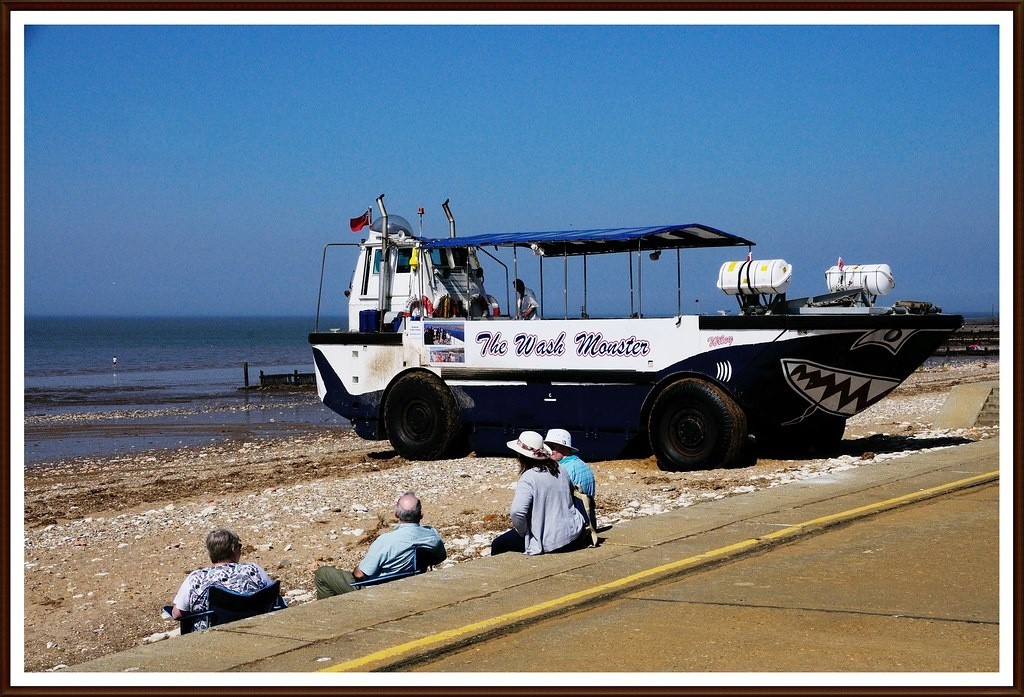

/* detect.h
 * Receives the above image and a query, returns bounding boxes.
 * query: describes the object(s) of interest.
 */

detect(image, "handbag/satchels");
[574,484,599,548]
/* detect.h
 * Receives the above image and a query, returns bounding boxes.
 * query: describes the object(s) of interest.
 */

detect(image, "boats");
[306,192,966,460]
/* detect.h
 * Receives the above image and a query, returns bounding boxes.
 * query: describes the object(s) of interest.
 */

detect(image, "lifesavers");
[404,294,433,319]
[465,293,500,317]
[432,293,458,319]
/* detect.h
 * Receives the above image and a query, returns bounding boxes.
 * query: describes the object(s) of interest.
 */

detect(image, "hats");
[506,431,552,459]
[543,429,579,452]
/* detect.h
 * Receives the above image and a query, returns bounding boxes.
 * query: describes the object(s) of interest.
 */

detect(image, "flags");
[349,210,369,232]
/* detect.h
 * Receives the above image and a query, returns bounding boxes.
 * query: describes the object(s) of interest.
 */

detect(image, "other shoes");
[163,606,173,619]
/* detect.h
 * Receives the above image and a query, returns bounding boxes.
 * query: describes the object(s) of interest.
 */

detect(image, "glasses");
[238,543,242,549]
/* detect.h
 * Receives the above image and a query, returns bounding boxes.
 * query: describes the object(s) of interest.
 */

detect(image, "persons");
[512,278,540,321]
[490,431,586,557]
[172,529,288,633]
[314,494,447,602]
[543,429,595,534]
